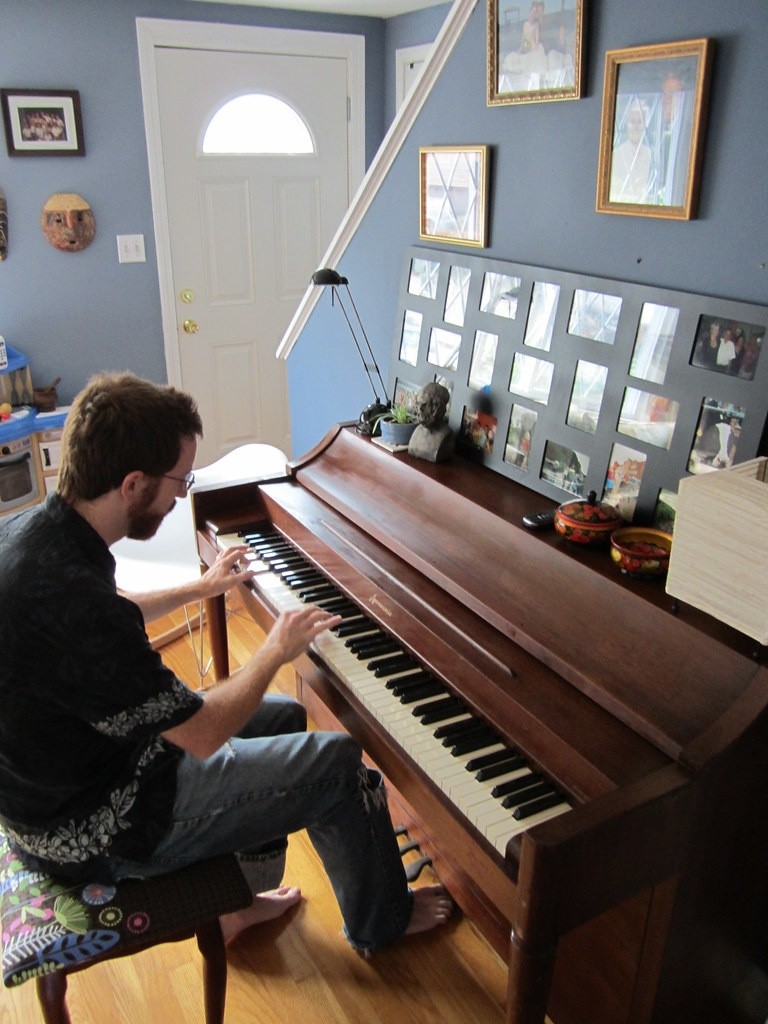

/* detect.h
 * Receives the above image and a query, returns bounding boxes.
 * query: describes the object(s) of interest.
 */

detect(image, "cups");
[33,387,56,412]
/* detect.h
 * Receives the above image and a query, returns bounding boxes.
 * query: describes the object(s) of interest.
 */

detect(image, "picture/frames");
[0,88,86,156]
[418,146,489,248]
[596,38,709,221]
[486,0,583,107]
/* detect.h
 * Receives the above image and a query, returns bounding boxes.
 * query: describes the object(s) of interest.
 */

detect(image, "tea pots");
[356,397,392,435]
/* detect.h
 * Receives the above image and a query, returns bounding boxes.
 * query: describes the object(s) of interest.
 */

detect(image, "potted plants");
[368,388,423,446]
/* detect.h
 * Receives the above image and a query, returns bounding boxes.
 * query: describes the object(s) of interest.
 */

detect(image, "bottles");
[0,335,8,370]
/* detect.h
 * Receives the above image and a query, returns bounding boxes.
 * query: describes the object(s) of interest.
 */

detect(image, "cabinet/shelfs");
[0,347,72,520]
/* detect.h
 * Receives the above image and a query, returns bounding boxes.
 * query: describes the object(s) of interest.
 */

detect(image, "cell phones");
[522,509,557,528]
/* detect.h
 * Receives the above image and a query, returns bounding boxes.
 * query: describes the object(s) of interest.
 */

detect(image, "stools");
[0,823,253,1024]
[108,442,289,696]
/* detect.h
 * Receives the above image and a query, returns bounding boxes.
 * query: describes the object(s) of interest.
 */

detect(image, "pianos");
[185,411,768,1023]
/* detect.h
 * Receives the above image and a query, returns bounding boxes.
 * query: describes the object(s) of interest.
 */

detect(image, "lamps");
[312,268,391,435]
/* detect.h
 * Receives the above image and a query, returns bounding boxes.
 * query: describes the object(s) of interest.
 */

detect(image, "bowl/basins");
[555,490,620,543]
[610,527,672,573]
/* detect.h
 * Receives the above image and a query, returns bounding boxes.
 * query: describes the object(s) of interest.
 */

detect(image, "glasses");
[141,470,195,490]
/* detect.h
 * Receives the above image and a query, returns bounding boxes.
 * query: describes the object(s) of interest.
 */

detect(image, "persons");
[0,373,451,955]
[610,75,688,207]
[514,431,530,466]
[698,325,762,379]
[472,422,490,454]
[501,0,545,72]
[699,417,740,468]
[559,459,576,493]
[21,110,64,140]
